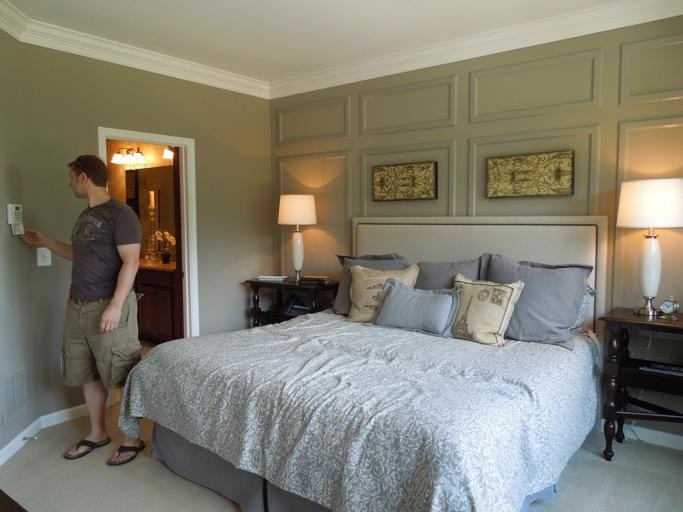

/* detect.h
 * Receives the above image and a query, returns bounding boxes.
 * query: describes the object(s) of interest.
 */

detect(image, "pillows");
[415,253,488,290]
[366,276,463,341]
[571,285,598,335]
[449,274,524,347]
[479,252,593,352]
[334,252,412,318]
[347,265,421,324]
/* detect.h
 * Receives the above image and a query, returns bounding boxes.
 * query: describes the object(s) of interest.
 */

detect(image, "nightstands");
[247,278,338,329]
[597,307,682,461]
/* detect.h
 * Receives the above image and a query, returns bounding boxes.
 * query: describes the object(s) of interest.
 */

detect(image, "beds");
[118,215,609,512]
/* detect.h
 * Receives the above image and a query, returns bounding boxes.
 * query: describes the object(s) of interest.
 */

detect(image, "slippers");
[63,435,110,459]
[106,439,145,465]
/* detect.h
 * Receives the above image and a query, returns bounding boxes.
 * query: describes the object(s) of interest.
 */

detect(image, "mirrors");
[125,165,174,262]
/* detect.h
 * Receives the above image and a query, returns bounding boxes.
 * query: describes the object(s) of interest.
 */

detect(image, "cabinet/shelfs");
[134,268,182,342]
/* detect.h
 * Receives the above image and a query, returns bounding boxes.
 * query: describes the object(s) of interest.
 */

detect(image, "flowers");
[154,230,176,251]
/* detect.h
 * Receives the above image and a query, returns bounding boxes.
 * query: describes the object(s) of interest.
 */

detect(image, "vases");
[162,251,170,265]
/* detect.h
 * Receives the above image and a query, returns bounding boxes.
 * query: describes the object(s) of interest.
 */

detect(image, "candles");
[149,191,156,207]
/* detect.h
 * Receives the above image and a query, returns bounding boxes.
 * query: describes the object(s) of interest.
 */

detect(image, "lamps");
[277,194,317,281]
[111,143,142,165]
[614,178,683,319]
[162,147,175,162]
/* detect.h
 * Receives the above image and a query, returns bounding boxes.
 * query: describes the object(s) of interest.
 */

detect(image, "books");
[299,275,331,286]
[637,360,683,377]
[256,274,289,282]
[289,305,312,316]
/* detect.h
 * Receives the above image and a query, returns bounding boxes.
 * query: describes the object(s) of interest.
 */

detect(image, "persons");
[19,154,145,465]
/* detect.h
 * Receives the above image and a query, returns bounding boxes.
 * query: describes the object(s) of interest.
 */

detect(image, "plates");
[256,275,288,282]
